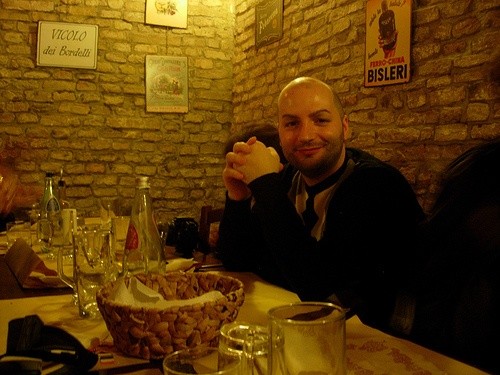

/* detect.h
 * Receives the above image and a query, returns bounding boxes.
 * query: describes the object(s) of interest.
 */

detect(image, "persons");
[209,123,289,270]
[218,77,429,338]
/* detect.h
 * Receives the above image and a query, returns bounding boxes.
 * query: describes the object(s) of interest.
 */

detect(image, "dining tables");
[0,223,491,375]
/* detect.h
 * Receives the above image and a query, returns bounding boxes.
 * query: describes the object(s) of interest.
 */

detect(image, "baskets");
[96,272,245,360]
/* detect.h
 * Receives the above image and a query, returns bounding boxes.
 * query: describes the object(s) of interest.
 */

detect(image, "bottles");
[40,172,63,253]
[58,181,78,224]
[123,176,167,278]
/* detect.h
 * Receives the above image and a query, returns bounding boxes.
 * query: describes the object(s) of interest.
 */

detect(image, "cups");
[54,226,70,245]
[6,221,33,250]
[57,224,113,320]
[109,215,130,261]
[217,318,288,375]
[53,208,77,246]
[266,301,346,375]
[163,345,243,375]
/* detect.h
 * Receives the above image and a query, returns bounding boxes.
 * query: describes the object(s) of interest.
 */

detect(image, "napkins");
[109,277,223,307]
[344,337,448,375]
[164,258,197,272]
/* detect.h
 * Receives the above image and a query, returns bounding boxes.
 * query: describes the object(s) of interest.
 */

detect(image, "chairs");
[408,141,500,375]
